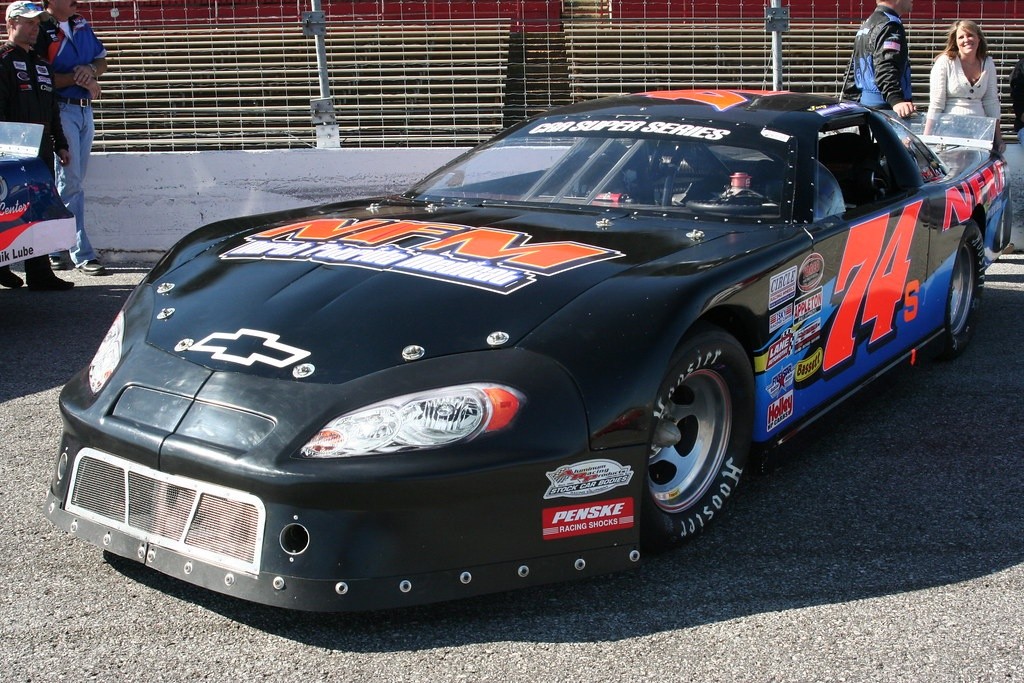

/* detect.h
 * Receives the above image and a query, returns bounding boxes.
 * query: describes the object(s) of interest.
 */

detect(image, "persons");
[1010,56,1024,150]
[852,0,917,130]
[40,0,106,276]
[923,21,1006,191]
[0,0,74,293]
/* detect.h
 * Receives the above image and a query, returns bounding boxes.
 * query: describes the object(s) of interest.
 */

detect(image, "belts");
[57,96,91,107]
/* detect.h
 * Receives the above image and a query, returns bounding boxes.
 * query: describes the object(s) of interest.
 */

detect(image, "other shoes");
[1003,242,1014,254]
[26,270,74,290]
[0,266,24,288]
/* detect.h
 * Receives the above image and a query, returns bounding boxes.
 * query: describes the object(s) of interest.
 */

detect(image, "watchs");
[88,64,97,73]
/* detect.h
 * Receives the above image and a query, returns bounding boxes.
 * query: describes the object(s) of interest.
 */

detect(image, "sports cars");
[40,81,1014,614]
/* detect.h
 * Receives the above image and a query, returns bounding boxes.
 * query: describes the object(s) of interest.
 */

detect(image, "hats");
[5,1,48,22]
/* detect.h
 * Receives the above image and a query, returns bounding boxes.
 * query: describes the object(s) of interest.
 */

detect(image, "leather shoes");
[48,256,67,270]
[78,261,105,275]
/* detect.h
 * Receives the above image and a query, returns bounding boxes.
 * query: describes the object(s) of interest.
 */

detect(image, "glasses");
[9,3,43,18]
